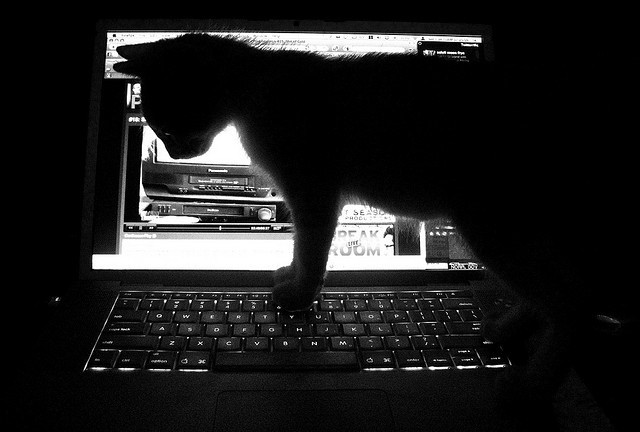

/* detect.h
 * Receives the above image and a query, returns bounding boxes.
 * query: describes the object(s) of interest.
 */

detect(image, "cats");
[107,31,599,372]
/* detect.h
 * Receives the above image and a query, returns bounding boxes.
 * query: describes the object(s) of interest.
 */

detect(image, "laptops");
[6,15,621,431]
